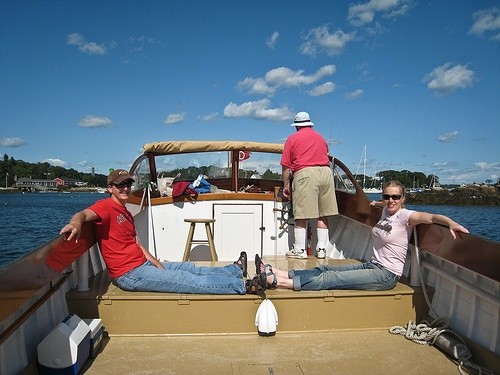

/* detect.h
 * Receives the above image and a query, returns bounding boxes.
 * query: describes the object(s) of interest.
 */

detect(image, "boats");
[97,190,105,194]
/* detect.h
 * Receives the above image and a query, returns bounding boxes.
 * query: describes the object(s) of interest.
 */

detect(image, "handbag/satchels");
[189,175,212,194]
[158,171,182,198]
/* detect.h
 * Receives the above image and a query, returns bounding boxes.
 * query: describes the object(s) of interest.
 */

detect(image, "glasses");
[383,194,402,200]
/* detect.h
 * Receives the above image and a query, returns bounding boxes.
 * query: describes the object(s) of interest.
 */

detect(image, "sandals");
[244,272,267,294]
[233,251,248,277]
[255,253,277,289]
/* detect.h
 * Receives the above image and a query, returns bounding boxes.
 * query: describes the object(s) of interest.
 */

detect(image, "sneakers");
[314,248,326,259]
[286,244,308,259]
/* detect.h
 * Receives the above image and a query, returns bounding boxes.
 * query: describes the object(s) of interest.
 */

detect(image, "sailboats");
[353,143,384,194]
[407,173,440,193]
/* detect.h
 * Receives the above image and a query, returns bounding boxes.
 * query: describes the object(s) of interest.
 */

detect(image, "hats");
[290,111,314,127]
[106,169,136,186]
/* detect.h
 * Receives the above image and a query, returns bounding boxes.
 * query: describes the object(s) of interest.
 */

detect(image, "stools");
[182,219,217,262]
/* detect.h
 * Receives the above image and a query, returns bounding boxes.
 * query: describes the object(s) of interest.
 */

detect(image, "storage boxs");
[81,318,105,359]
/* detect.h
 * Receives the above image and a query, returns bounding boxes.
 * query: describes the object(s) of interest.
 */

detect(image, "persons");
[255,180,470,291]
[59,169,266,295]
[280,111,339,259]
